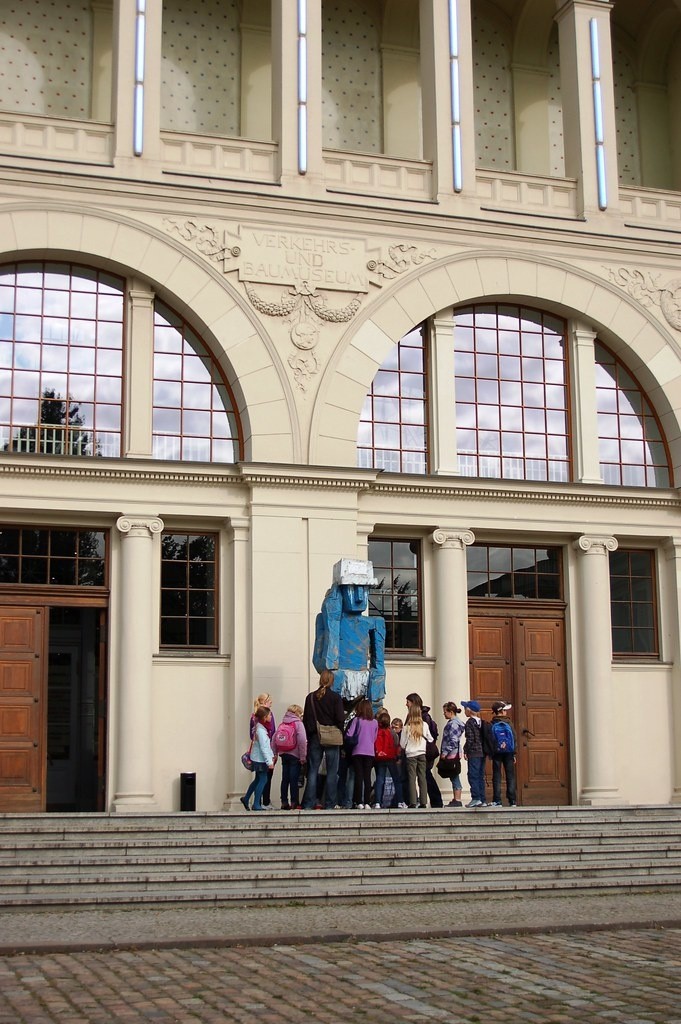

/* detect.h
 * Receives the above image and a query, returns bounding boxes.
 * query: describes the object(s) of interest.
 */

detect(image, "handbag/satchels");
[346,718,359,747]
[436,757,461,777]
[242,752,253,771]
[316,722,343,746]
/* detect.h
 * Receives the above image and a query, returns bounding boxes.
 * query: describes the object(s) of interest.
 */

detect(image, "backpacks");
[480,719,494,756]
[274,721,297,751]
[491,721,514,755]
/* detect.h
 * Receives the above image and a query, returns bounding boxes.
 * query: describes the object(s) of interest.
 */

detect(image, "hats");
[461,700,480,712]
[492,702,512,713]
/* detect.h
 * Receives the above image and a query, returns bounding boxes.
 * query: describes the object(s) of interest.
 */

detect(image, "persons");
[250,693,276,810]
[240,707,275,810]
[461,701,487,807]
[488,701,518,807]
[436,702,466,808]
[272,704,307,809]
[298,692,443,809]
[304,670,344,810]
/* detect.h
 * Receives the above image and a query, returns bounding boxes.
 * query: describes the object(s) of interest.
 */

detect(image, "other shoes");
[510,800,516,807]
[281,801,372,809]
[252,805,265,810]
[240,797,250,811]
[398,802,408,808]
[418,804,426,808]
[375,803,381,808]
[407,804,415,808]
[487,801,502,808]
[444,800,462,808]
[261,804,278,810]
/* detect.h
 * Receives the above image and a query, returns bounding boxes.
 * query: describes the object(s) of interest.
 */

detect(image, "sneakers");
[465,800,482,807]
[477,802,487,807]
